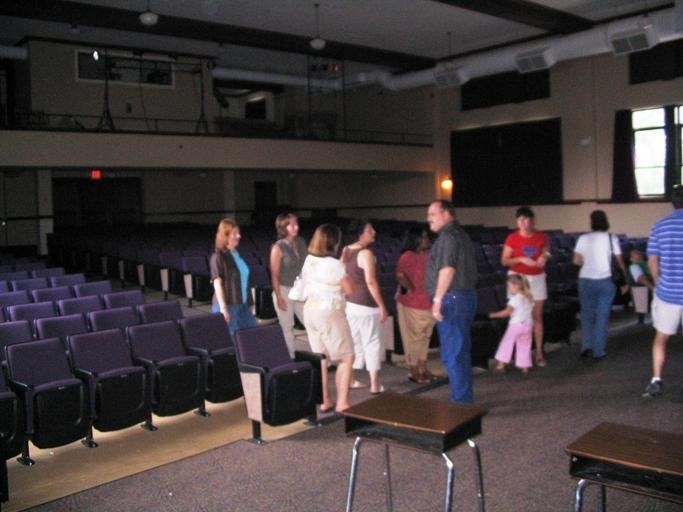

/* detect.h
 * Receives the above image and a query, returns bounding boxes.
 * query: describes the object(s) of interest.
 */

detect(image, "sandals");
[492,356,547,377]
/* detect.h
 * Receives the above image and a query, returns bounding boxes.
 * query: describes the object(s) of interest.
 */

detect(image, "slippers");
[370,382,390,395]
[349,379,368,390]
[410,368,438,384]
[318,401,337,414]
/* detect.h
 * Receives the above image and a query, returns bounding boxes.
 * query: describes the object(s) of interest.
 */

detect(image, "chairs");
[0,280,8,291]
[100,289,145,310]
[0,307,5,323]
[176,312,244,417]
[30,286,72,302]
[0,365,20,461]
[0,290,30,308]
[32,313,88,339]
[377,286,436,352]
[0,320,33,360]
[179,255,212,307]
[549,283,577,342]
[9,277,47,292]
[0,264,13,272]
[136,300,183,324]
[54,295,103,315]
[7,300,56,321]
[3,338,87,467]
[235,266,275,318]
[124,320,201,431]
[0,271,28,280]
[86,305,139,331]
[66,327,145,448]
[468,287,508,362]
[32,220,649,298]
[13,262,46,271]
[231,323,326,445]
[492,283,552,350]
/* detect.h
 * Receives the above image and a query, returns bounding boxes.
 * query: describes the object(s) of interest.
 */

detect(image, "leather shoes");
[582,350,609,360]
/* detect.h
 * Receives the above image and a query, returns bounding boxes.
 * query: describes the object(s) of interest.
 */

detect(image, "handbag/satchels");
[288,272,309,303]
[609,232,626,284]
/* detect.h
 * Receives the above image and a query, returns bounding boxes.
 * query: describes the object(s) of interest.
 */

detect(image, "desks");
[342,389,488,512]
[563,421,682,512]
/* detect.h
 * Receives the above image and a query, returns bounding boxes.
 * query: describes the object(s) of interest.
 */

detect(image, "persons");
[340,221,388,396]
[500,205,548,368]
[396,221,434,385]
[425,197,478,406]
[289,224,354,415]
[643,186,683,400]
[268,213,309,356]
[208,216,256,340]
[488,274,534,375]
[573,209,629,360]
[629,249,651,323]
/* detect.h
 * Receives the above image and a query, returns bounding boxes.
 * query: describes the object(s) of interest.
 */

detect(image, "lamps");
[308,4,325,49]
[137,0,157,25]
[441,179,452,189]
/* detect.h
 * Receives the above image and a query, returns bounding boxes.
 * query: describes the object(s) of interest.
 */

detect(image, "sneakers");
[642,381,663,397]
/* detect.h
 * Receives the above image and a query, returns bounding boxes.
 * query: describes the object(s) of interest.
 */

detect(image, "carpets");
[70,321,682,512]
